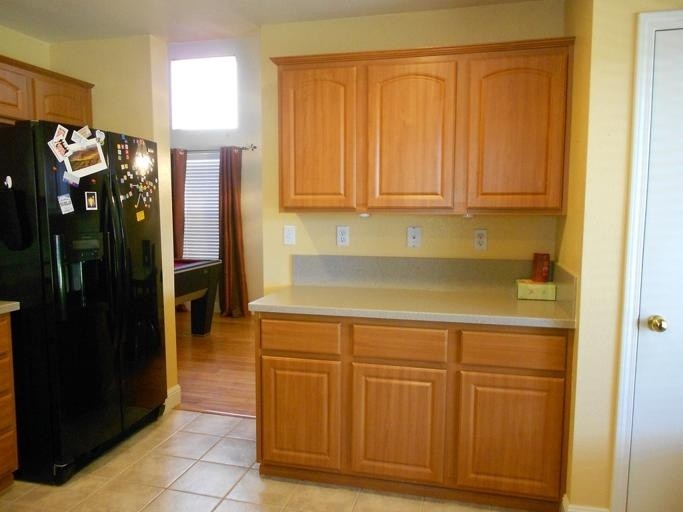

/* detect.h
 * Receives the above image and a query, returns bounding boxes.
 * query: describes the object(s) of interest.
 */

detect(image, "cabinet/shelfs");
[2,55,95,128]
[0,316,22,494]
[255,312,574,510]
[268,38,572,214]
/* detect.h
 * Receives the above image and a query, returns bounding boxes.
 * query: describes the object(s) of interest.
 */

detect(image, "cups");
[530,252,551,283]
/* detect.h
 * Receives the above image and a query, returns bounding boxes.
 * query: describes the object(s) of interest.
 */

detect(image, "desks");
[174,258,224,339]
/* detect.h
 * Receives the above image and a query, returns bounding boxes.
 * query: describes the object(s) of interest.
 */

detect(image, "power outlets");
[473,229,488,252]
[335,226,349,246]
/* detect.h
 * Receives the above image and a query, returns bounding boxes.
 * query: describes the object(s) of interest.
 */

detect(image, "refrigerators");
[0,120,166,486]
